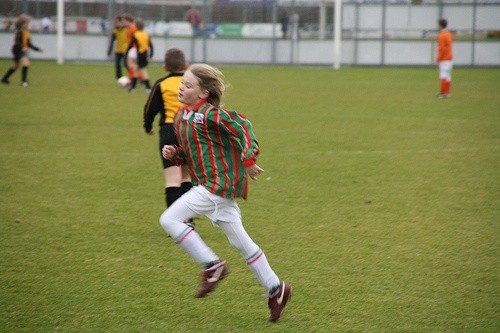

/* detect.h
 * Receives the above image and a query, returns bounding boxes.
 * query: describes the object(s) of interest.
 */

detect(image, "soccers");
[117,76,131,89]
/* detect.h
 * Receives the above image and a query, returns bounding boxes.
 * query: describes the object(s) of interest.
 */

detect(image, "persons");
[159,62,295,323]
[101,13,154,92]
[1,13,43,87]
[184,2,202,34]
[0,12,51,33]
[435,17,456,96]
[143,45,199,231]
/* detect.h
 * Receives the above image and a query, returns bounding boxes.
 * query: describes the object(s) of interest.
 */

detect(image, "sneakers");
[195,261,228,298]
[267,282,291,321]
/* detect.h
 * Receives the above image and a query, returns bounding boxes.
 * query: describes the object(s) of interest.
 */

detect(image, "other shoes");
[127,88,135,92]
[20,82,28,87]
[1,78,10,84]
[145,88,152,94]
